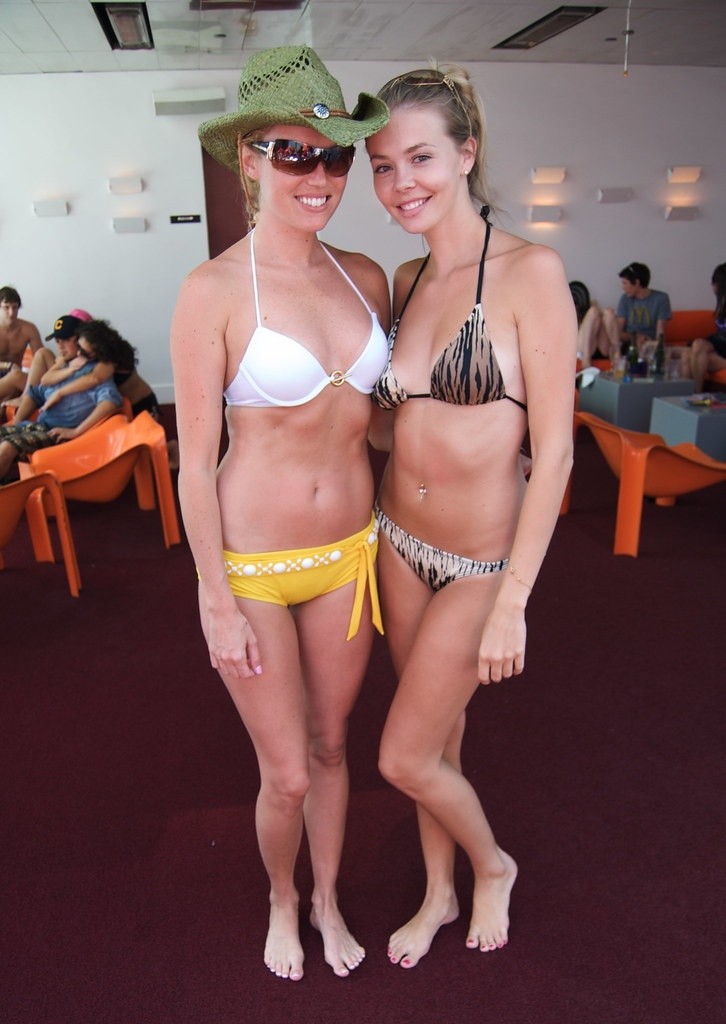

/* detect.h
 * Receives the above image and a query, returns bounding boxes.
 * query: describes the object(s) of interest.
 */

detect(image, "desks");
[649,394,726,463]
[579,370,695,434]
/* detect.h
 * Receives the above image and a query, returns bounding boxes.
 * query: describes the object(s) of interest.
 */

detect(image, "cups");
[612,355,626,379]
[668,362,681,380]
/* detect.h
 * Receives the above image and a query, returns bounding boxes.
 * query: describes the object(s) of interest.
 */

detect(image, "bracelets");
[4,360,8,371]
[506,559,535,593]
[8,361,14,371]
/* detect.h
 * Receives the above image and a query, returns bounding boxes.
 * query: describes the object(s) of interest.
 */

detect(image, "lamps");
[527,204,562,223]
[112,217,147,234]
[530,166,567,185]
[153,87,227,116]
[597,188,634,203]
[664,206,699,222]
[33,199,70,218]
[666,165,703,184]
[107,176,144,195]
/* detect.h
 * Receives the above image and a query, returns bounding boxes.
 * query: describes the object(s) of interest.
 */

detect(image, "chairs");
[0,348,133,463]
[18,410,180,564]
[559,412,726,558]
[0,471,82,598]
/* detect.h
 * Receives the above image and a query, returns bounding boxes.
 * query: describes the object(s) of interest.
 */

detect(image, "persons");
[569,279,622,371]
[168,44,533,982]
[615,262,671,374]
[0,286,180,484]
[362,69,579,970]
[679,262,725,395]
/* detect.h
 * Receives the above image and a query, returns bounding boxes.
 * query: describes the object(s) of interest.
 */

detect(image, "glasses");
[76,342,94,360]
[376,68,472,144]
[247,139,356,177]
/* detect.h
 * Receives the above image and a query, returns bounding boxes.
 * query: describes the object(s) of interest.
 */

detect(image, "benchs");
[575,310,726,384]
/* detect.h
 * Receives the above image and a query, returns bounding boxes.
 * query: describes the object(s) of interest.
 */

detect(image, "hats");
[197,44,389,176]
[45,315,86,342]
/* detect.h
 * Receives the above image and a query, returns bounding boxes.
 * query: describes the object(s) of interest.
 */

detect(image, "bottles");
[650,334,664,375]
[627,332,639,370]
